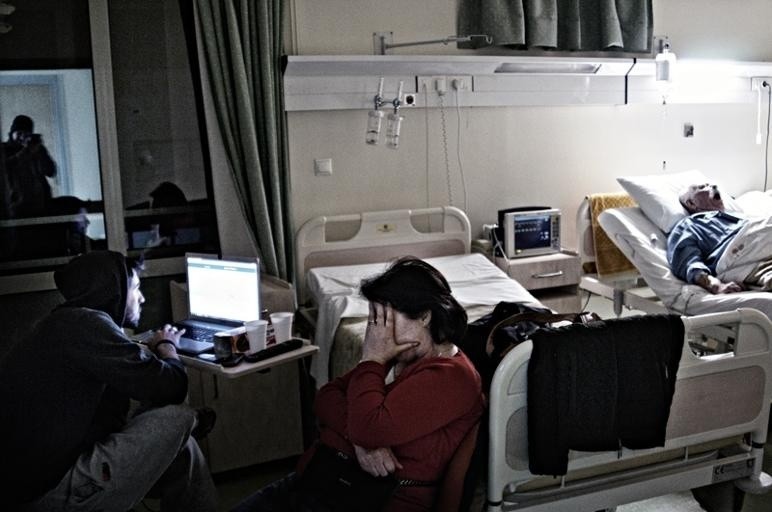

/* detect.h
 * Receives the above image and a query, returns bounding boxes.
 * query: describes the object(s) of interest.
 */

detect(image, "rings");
[369,321,376,326]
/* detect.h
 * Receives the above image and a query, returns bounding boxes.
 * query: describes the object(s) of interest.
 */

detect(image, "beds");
[293,205,772,512]
[575,194,772,350]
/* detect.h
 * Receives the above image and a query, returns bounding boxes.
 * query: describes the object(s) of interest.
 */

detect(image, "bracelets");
[154,340,176,353]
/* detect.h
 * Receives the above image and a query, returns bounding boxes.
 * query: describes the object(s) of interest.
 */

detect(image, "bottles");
[366,110,404,150]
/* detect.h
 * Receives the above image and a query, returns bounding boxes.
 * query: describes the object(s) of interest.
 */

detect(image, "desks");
[130,314,321,474]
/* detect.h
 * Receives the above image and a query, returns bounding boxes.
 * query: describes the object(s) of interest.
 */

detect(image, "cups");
[268,309,293,343]
[244,320,268,351]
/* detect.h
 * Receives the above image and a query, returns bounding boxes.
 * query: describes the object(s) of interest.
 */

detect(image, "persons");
[0,115,56,219]
[666,181,772,294]
[244,255,488,512]
[53,196,90,249]
[0,250,221,512]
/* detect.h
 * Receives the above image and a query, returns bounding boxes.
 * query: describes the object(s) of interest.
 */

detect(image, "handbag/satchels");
[294,443,395,512]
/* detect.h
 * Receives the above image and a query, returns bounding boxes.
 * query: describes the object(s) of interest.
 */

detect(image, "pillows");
[617,174,744,234]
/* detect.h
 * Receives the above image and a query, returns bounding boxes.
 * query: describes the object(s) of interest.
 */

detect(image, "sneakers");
[191,406,218,439]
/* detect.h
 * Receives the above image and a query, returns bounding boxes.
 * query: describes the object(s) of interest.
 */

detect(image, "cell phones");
[221,353,245,367]
[197,352,225,363]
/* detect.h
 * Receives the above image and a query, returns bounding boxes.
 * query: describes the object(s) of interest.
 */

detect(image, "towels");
[583,194,643,278]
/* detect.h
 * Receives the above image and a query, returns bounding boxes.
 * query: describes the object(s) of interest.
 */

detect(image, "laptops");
[141,252,261,355]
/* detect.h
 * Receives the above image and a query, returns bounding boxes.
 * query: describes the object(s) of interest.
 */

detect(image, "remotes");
[246,339,303,363]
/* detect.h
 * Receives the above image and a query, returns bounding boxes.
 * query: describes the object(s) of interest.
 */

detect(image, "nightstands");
[477,245,582,316]
[169,273,299,324]
